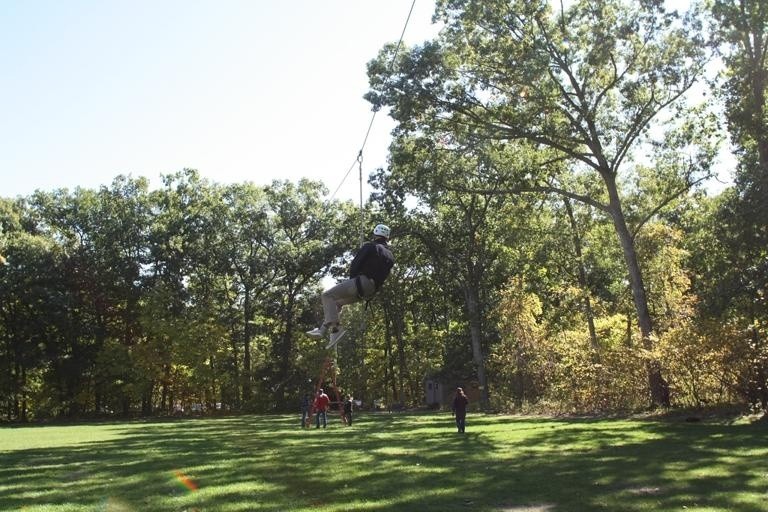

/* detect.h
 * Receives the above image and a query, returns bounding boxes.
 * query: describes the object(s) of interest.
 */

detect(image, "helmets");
[373,224,390,239]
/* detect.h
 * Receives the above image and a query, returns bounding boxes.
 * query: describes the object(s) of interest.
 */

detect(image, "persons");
[388,399,405,413]
[304,224,394,350]
[300,388,354,428]
[451,388,470,435]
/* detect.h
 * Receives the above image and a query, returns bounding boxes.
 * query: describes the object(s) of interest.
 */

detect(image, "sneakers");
[305,327,325,339]
[325,328,349,349]
